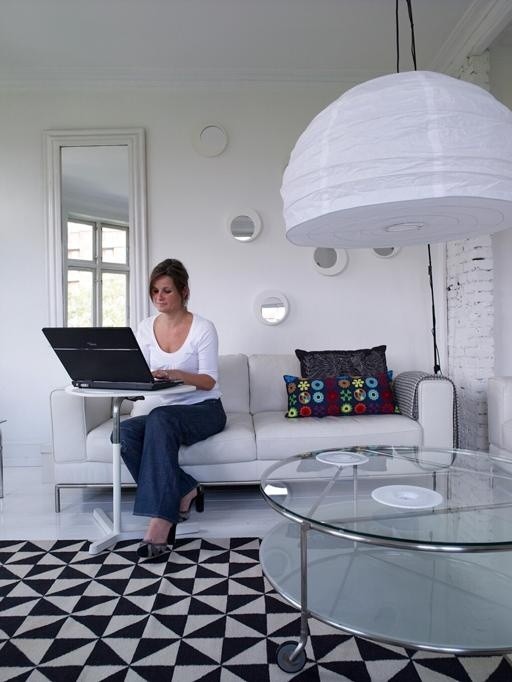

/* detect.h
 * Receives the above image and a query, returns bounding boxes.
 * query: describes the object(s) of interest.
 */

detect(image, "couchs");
[38,353,464,514]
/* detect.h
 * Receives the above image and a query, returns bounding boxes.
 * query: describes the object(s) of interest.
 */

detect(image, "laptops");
[42,327,184,390]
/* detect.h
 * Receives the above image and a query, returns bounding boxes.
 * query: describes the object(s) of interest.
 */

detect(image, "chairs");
[483,377,510,490]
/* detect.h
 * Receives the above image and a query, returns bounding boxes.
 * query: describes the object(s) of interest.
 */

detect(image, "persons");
[111,259,226,559]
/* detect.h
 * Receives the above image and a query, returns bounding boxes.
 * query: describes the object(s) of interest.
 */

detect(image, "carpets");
[1,542,512,679]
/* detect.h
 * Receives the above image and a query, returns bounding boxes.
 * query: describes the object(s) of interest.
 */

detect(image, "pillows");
[284,342,400,416]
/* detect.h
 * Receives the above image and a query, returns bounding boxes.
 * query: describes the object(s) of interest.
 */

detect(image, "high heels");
[176,486,205,523]
[136,519,177,557]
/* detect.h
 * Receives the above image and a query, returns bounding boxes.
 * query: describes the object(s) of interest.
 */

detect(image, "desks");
[63,381,201,555]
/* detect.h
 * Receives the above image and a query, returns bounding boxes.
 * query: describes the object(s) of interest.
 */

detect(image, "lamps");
[276,0,512,251]
